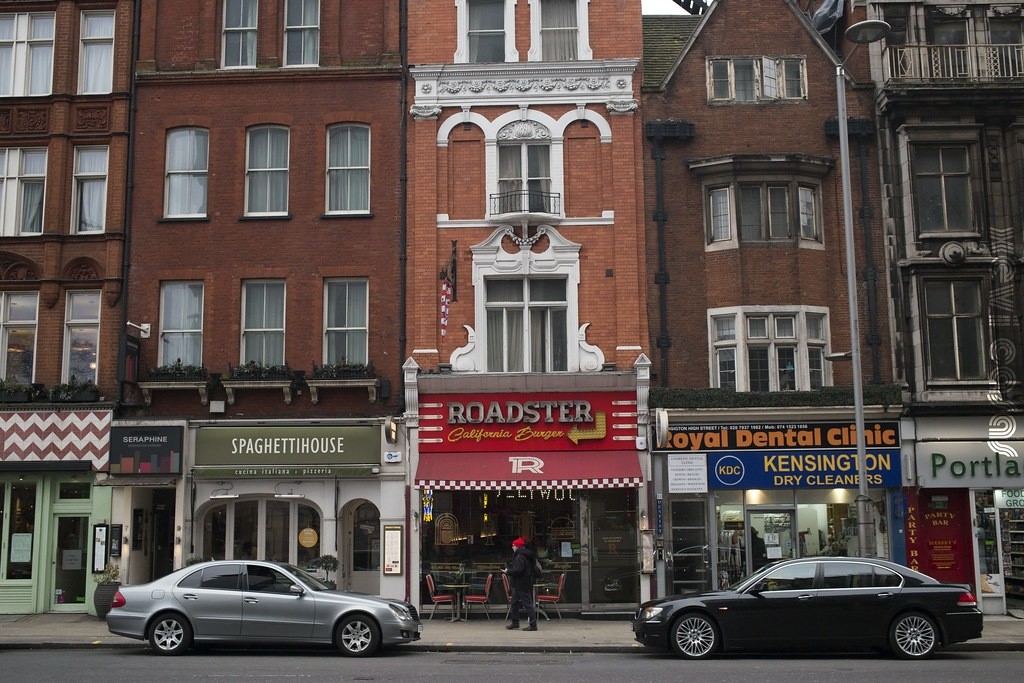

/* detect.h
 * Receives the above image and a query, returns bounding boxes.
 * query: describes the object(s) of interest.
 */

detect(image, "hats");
[512,537,525,548]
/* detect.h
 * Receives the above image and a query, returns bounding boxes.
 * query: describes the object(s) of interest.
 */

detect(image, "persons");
[730,523,745,580]
[734,527,766,583]
[500,538,537,631]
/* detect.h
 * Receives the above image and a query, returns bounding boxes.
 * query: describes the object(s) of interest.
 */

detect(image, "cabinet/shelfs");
[1004,519,1024,596]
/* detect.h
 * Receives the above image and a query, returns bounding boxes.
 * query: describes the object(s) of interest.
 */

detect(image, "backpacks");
[530,555,542,579]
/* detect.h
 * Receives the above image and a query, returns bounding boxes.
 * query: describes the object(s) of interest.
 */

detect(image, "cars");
[106,558,423,656]
[307,548,380,572]
[603,545,746,602]
[632,556,984,661]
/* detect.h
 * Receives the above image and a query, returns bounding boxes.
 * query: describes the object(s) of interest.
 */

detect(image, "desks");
[439,583,473,622]
[532,583,551,621]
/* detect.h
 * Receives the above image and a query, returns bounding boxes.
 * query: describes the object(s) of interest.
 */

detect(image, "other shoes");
[522,624,538,632]
[505,622,520,629]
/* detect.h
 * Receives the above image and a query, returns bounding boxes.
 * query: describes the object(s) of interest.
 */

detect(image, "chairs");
[426,574,456,623]
[465,574,493,623]
[502,574,531,622]
[536,574,565,621]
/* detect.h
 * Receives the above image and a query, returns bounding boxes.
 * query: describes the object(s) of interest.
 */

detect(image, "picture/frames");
[109,524,123,558]
[92,524,109,574]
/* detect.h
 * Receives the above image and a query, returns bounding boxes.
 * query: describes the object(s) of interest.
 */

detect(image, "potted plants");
[146,357,208,381]
[93,564,121,622]
[312,353,377,379]
[228,360,292,381]
[0,374,100,403]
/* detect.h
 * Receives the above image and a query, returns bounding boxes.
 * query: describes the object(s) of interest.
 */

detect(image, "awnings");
[414,451,643,490]
